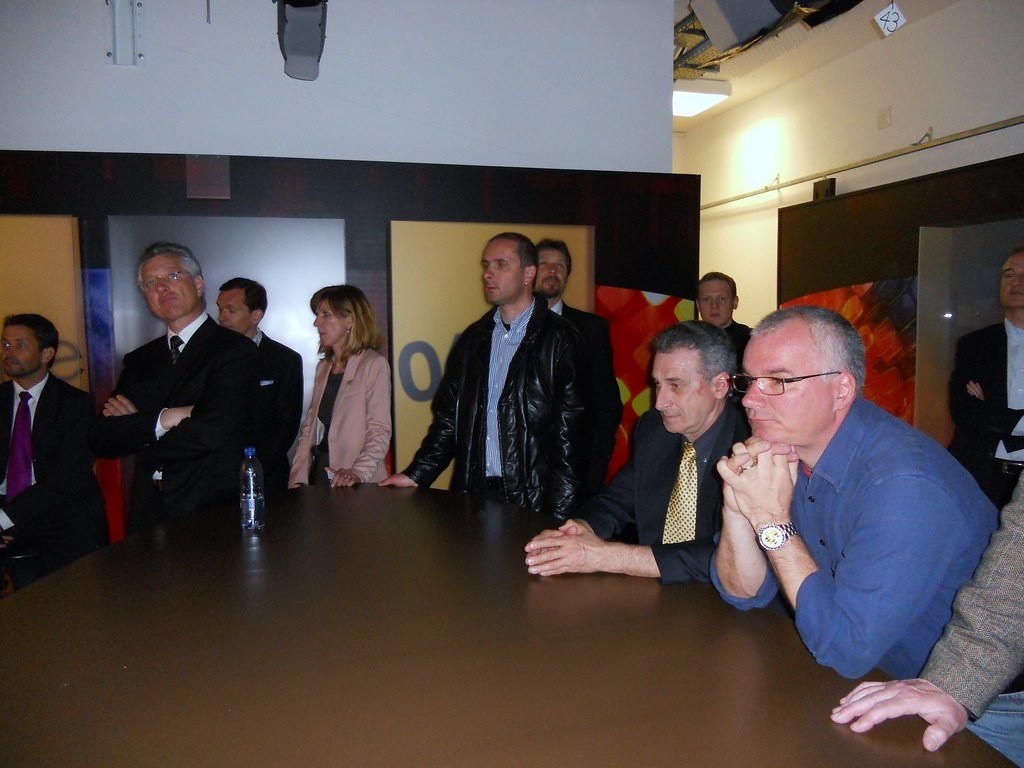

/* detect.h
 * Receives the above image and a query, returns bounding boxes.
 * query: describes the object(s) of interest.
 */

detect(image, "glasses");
[139,270,190,291]
[1,341,44,351]
[732,372,839,395]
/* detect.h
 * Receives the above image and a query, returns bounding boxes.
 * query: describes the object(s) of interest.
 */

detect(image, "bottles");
[239,448,265,532]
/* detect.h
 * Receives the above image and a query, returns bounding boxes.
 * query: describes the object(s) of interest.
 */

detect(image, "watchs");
[755,523,798,552]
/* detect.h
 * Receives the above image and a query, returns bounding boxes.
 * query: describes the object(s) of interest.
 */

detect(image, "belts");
[989,458,1024,476]
[485,475,504,491]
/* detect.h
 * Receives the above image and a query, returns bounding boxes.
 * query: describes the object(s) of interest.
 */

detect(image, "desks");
[0,484,1021,768]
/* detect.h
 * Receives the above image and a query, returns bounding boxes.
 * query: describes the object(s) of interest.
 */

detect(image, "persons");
[216,276,303,479]
[288,283,392,488]
[532,237,624,498]
[0,313,111,591]
[831,465,1024,752]
[524,316,751,586]
[944,247,1024,510]
[377,232,605,522]
[709,302,1024,768]
[93,240,264,521]
[696,271,752,372]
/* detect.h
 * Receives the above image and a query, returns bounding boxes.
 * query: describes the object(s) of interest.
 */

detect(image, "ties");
[662,442,697,544]
[169,336,184,365]
[6,391,32,502]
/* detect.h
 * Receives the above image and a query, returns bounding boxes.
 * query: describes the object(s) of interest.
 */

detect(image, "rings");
[346,475,352,480]
[742,462,755,470]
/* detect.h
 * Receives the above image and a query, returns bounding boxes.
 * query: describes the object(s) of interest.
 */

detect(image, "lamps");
[670,77,733,119]
